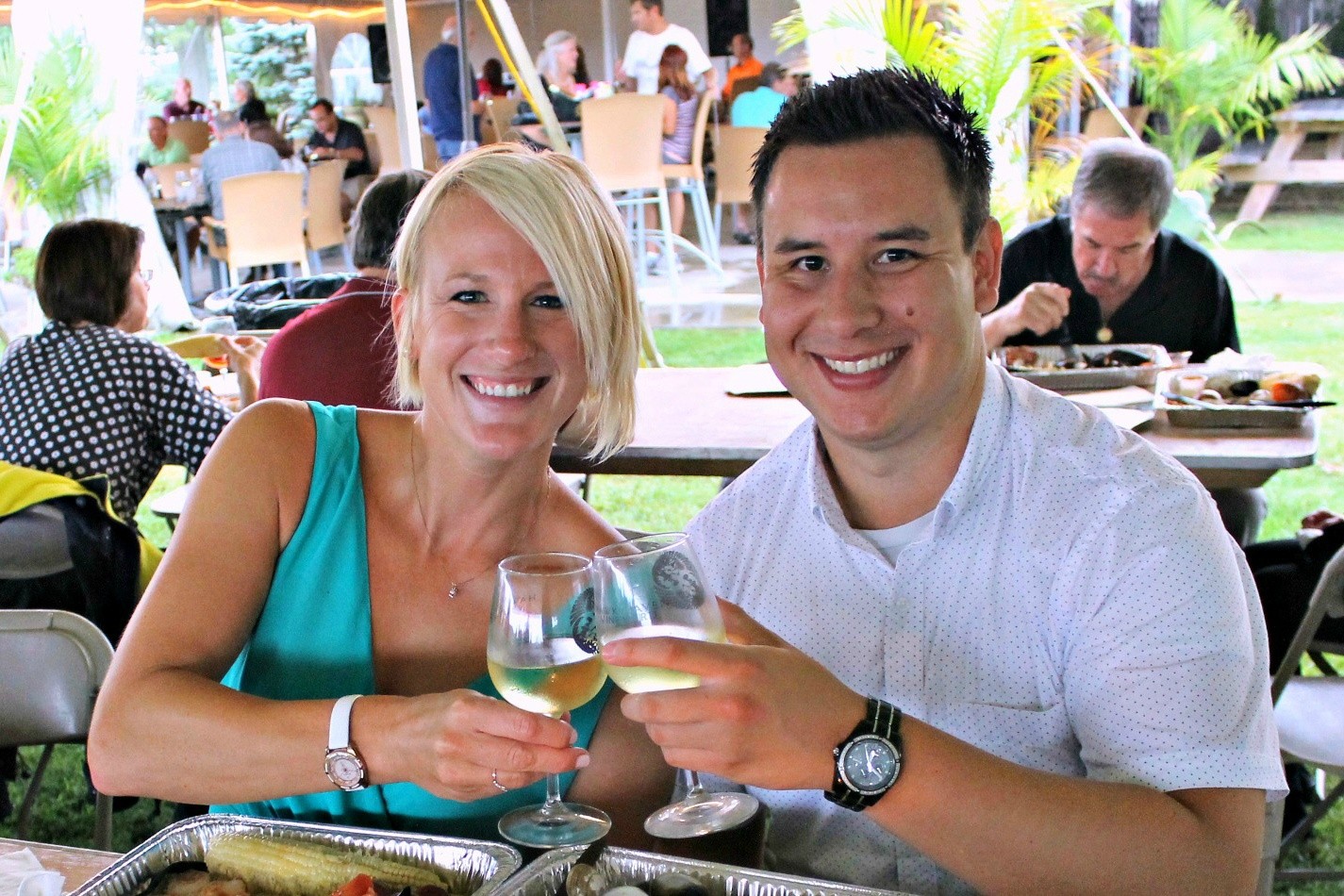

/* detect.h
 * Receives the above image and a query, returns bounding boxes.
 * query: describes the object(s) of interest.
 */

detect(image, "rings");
[492,769,509,793]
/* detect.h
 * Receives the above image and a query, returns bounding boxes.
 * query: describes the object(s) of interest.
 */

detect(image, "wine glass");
[594,532,760,840]
[304,144,319,169]
[485,555,611,850]
[201,316,236,373]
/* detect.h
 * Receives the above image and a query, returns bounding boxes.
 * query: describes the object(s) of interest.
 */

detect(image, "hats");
[240,99,271,123]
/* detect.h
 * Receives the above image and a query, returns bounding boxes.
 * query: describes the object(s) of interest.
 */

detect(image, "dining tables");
[545,363,1319,507]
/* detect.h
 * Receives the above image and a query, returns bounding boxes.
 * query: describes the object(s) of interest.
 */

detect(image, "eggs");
[1171,374,1207,395]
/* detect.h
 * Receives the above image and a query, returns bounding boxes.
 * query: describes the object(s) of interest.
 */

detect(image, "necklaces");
[409,408,552,598]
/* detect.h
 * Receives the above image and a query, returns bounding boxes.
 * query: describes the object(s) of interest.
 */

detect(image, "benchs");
[1234,100,1344,222]
[1216,141,1271,226]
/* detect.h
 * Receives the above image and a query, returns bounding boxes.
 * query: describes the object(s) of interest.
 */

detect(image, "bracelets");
[335,149,341,161]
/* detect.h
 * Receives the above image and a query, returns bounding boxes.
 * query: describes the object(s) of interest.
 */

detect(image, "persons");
[416,0,814,276]
[1242,509,1344,839]
[260,170,432,414]
[665,68,1289,896]
[0,217,267,815]
[133,78,372,285]
[980,138,1268,548]
[87,141,676,868]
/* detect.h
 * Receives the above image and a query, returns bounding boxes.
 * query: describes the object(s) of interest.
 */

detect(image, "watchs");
[324,694,368,793]
[825,697,903,811]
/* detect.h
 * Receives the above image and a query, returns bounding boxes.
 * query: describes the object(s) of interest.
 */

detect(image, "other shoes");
[646,251,661,269]
[1281,762,1325,844]
[83,760,140,811]
[0,781,13,822]
[732,230,757,245]
[651,251,685,274]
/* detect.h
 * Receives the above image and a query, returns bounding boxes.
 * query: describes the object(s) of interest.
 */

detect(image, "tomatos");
[327,873,378,896]
[1273,382,1313,403]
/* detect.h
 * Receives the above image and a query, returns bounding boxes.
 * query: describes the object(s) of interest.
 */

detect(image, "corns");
[205,831,447,896]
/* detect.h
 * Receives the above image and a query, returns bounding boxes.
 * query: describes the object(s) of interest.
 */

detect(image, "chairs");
[201,156,350,291]
[1042,107,1149,158]
[357,95,517,181]
[0,459,165,852]
[579,88,772,281]
[1251,543,1344,896]
[146,119,211,193]
